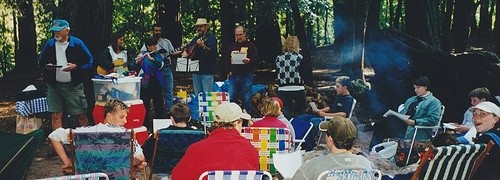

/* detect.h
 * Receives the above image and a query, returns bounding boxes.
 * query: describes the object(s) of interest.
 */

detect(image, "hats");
[213,102,251,122]
[319,116,356,143]
[468,101,500,117]
[49,19,69,31]
[194,18,209,25]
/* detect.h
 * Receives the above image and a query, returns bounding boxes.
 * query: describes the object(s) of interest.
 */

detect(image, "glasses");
[472,112,491,117]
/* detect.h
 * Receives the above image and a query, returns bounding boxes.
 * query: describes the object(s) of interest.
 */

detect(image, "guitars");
[183,33,209,59]
[96,57,135,76]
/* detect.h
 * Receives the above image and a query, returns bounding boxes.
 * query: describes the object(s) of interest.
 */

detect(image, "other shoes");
[46,145,66,158]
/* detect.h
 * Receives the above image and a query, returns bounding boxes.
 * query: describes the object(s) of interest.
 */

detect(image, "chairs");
[315,98,357,148]
[240,126,292,176]
[36,173,110,180]
[388,105,445,165]
[68,129,144,180]
[316,168,382,180]
[199,170,274,180]
[290,117,315,150]
[198,92,230,128]
[0,128,46,180]
[410,141,496,180]
[148,129,206,180]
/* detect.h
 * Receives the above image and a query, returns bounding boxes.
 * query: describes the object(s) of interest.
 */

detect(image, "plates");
[47,65,62,67]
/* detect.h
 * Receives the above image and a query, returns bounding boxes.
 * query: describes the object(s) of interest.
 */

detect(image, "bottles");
[177,87,182,92]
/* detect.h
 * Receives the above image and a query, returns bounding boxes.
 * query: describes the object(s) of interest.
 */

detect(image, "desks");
[16,97,50,135]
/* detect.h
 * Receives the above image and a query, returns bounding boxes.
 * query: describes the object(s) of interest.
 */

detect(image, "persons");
[472,102,500,180]
[128,38,166,123]
[381,133,460,180]
[48,99,145,180]
[249,98,295,153]
[275,35,306,121]
[301,76,353,152]
[171,102,259,180]
[141,103,194,161]
[292,116,373,180]
[181,18,217,100]
[98,32,129,76]
[40,19,94,156]
[225,26,258,127]
[369,75,441,153]
[456,88,492,144]
[139,24,182,118]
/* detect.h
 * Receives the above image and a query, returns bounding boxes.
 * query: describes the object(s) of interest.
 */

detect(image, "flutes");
[134,50,158,61]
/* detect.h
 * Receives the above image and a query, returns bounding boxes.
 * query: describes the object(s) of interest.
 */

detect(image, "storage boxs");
[92,77,142,103]
[127,125,148,145]
[93,99,145,129]
